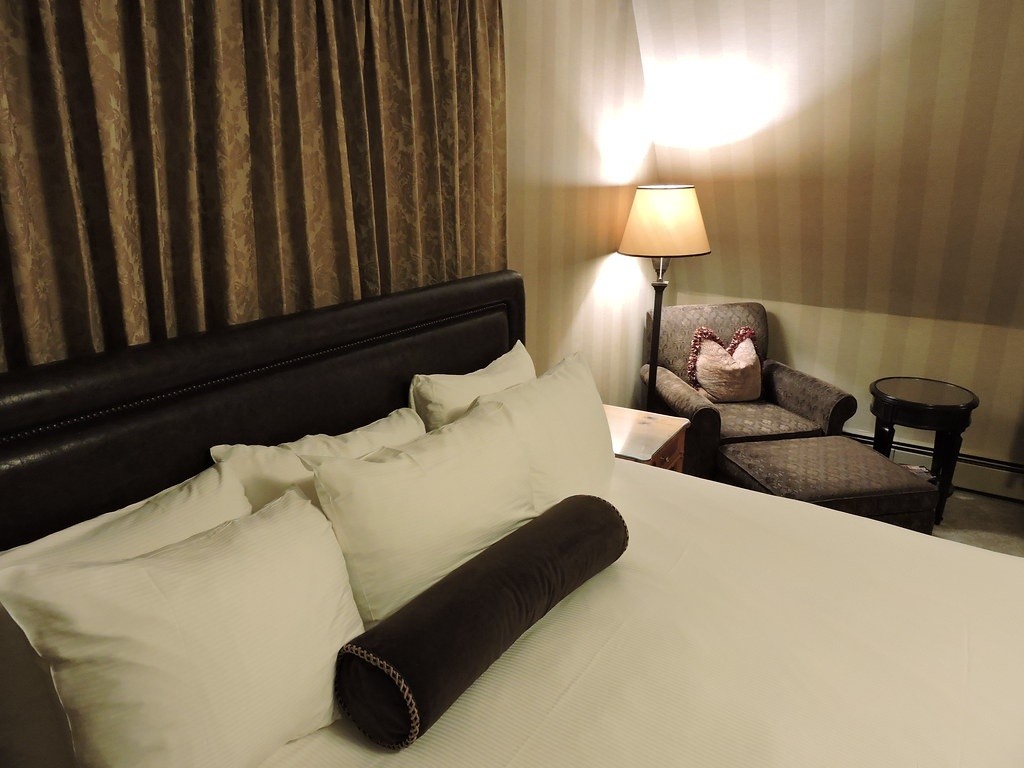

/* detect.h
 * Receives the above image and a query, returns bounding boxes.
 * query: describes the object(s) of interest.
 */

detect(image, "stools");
[869,377,980,527]
[714,435,940,535]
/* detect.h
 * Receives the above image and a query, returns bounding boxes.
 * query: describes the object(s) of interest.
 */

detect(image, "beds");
[0,271,1023,768]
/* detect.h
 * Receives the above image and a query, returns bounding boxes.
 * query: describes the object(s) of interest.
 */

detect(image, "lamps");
[616,184,712,414]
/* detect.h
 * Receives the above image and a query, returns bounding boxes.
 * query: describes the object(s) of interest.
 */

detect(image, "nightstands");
[603,404,692,473]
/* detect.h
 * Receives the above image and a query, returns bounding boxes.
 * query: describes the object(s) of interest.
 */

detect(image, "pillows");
[0,340,629,768]
[686,326,761,403]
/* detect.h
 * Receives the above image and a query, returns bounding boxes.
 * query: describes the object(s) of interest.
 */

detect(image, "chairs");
[639,302,858,484]
